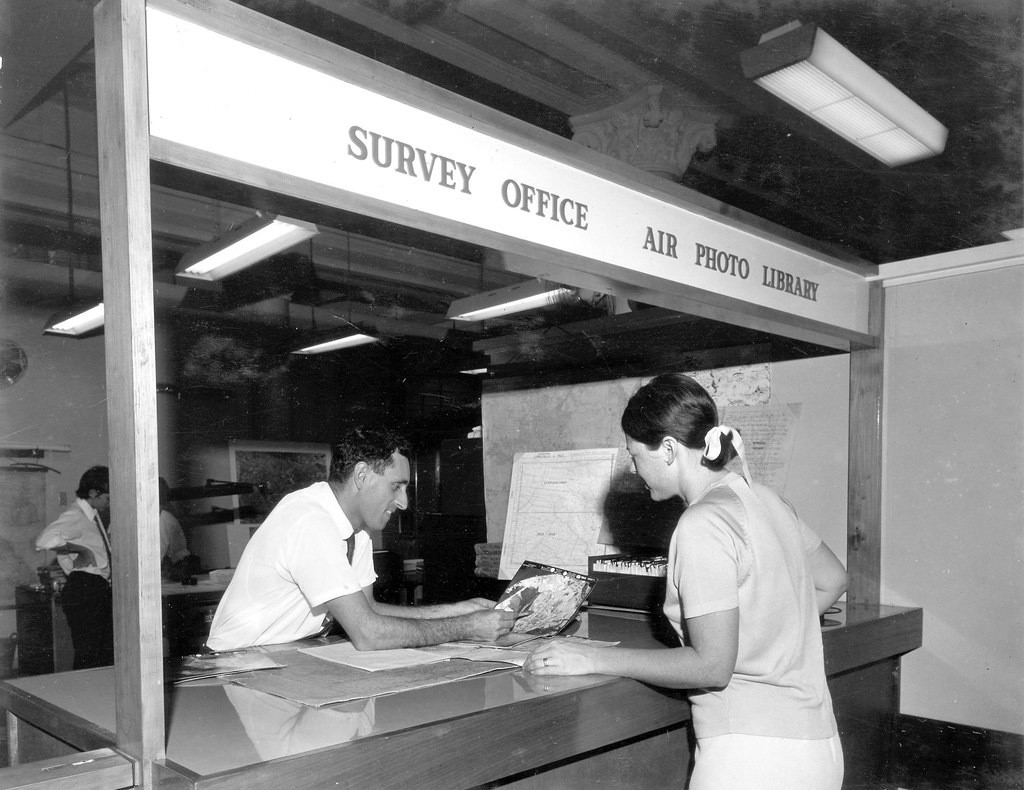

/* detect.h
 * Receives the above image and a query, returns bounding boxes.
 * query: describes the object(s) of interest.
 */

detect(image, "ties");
[294,533,355,642]
[94,516,112,581]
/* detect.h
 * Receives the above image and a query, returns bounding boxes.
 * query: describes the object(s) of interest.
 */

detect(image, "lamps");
[173,210,615,359]
[42,69,104,340]
[740,4,971,177]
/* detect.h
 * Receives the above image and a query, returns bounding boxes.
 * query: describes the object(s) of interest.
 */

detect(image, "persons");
[524,670,613,694]
[158,478,189,580]
[35,466,114,669]
[523,372,848,790]
[202,422,521,651]
[221,685,376,759]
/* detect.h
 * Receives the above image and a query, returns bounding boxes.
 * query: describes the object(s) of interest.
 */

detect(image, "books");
[465,560,598,648]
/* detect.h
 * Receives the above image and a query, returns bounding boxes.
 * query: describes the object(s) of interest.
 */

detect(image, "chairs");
[886,708,993,790]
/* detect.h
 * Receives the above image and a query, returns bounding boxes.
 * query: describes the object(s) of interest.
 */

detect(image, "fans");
[0,338,27,388]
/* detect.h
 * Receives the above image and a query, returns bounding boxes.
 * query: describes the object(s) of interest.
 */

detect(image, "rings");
[543,657,548,666]
[545,686,548,690]
